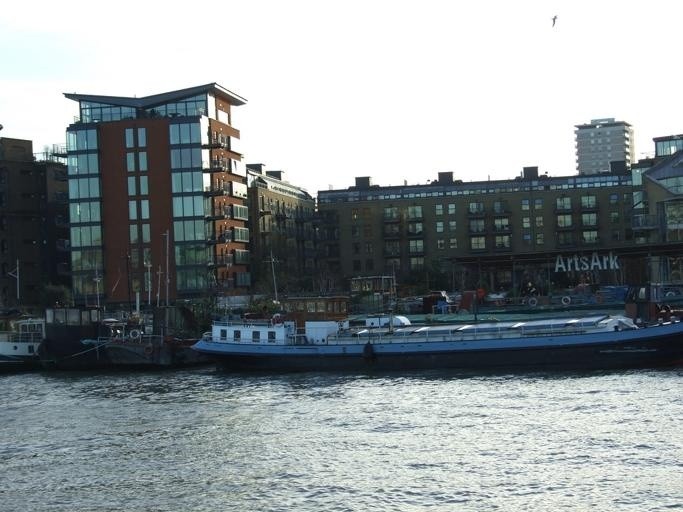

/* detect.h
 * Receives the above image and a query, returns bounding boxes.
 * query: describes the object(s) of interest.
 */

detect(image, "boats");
[190,295,682,375]
[0,297,213,373]
